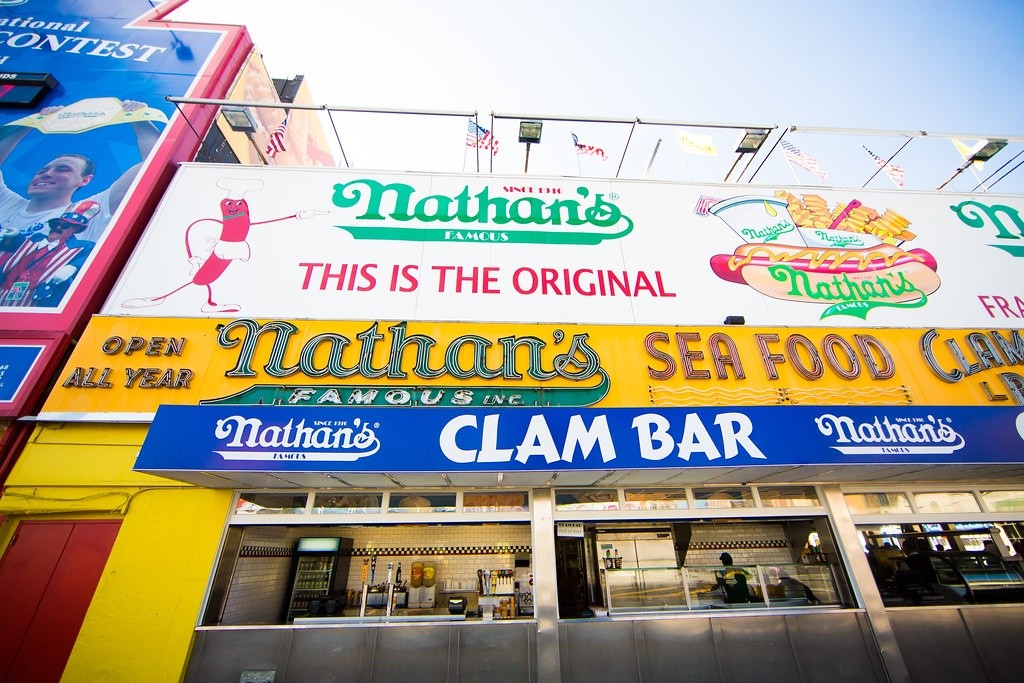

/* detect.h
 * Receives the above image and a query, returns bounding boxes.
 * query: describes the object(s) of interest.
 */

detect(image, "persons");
[776,570,822,606]
[864,539,1024,606]
[710,553,753,604]
[0,99,169,307]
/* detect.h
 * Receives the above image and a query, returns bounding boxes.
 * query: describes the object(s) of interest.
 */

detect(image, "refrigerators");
[286,537,354,625]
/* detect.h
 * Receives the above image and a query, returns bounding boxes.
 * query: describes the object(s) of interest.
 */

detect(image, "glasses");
[48,222,79,230]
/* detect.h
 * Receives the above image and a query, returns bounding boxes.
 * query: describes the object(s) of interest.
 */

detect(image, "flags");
[266,117,288,158]
[864,147,905,186]
[953,139,984,172]
[675,130,717,156]
[572,134,609,162]
[466,121,499,156]
[780,138,827,183]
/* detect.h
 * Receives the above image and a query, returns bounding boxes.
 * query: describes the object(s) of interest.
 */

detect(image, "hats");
[719,552,732,560]
[47,201,101,233]
[903,526,918,533]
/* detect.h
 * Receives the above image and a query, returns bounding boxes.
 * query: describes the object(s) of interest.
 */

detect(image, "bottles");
[607,549,613,569]
[809,545,827,562]
[396,562,402,583]
[524,591,528,605]
[615,548,620,568]
[289,557,332,622]
